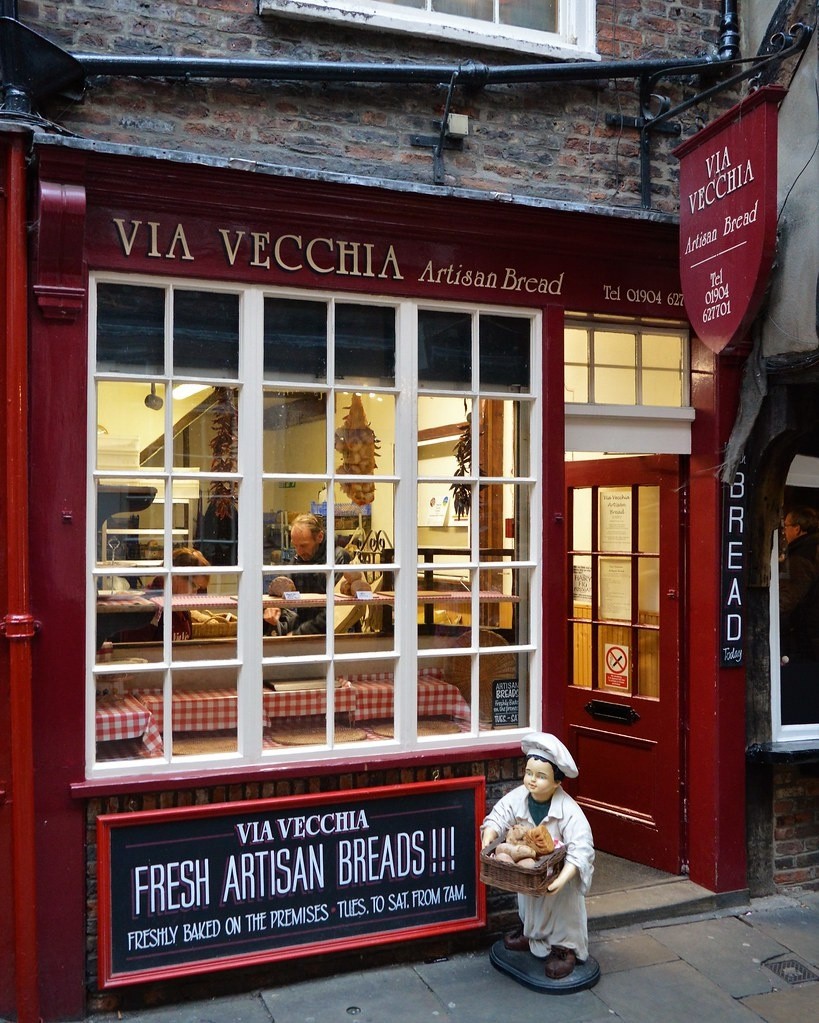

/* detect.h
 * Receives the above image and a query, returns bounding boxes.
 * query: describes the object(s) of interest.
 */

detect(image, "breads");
[144,540,159,558]
[495,826,555,869]
[340,572,371,597]
[189,610,218,624]
[268,576,297,597]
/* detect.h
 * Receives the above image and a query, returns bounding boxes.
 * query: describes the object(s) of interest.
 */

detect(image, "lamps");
[145,383,163,410]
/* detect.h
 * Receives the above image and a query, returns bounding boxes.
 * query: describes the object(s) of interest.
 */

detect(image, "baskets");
[189,609,237,637]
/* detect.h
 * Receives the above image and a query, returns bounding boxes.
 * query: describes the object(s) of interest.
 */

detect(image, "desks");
[95,675,470,757]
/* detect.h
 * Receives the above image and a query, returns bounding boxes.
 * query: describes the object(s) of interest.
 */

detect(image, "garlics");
[335,427,375,505]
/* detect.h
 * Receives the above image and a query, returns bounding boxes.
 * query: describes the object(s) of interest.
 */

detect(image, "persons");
[478,733,595,979]
[263,513,363,636]
[780,507,819,725]
[105,548,209,641]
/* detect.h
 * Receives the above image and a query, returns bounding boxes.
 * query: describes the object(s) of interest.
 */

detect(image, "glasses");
[192,582,201,591]
[782,523,798,530]
[297,517,320,527]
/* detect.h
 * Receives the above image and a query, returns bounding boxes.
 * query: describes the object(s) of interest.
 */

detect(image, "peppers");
[374,436,382,457]
[448,398,490,522]
[209,384,241,520]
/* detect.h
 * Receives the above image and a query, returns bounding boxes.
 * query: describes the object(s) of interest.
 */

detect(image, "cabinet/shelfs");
[280,511,363,565]
[105,501,194,595]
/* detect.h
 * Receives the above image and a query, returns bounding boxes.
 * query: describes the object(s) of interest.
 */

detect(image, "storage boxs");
[141,550,164,559]
[312,502,371,516]
[191,613,237,637]
